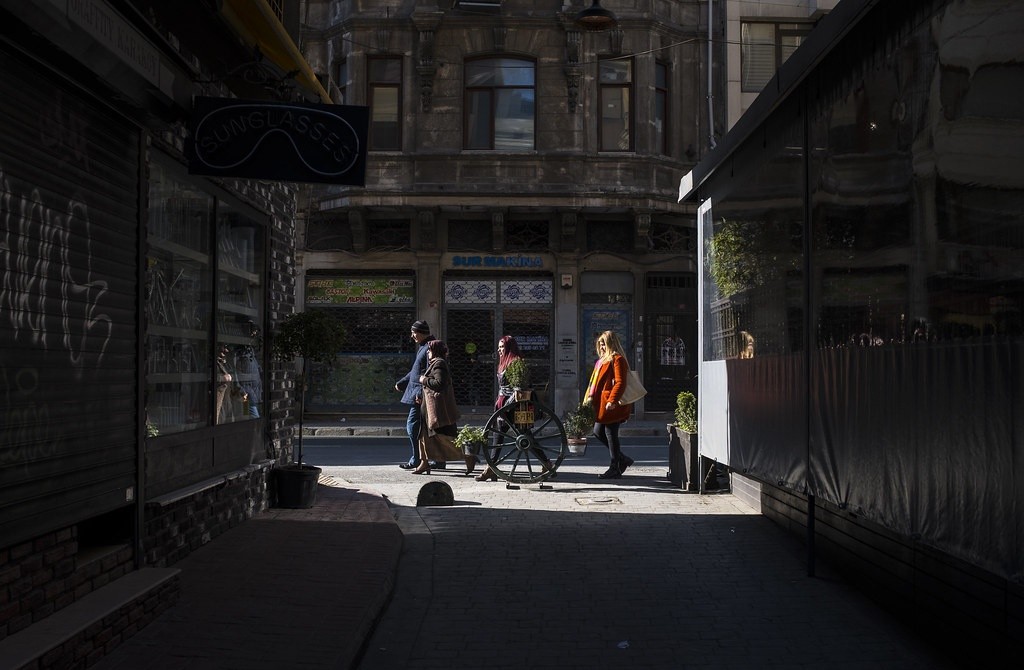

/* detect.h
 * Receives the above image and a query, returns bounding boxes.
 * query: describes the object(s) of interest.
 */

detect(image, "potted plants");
[269,309,348,508]
[450,424,488,456]
[703,217,791,358]
[562,415,594,456]
[666,390,729,491]
[505,359,534,402]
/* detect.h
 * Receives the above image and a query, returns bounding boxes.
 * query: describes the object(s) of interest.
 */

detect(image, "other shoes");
[598,465,622,479]
[398,462,416,469]
[620,451,634,474]
[430,463,445,469]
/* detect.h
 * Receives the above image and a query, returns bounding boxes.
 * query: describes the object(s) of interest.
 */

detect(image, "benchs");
[0,567,182,670]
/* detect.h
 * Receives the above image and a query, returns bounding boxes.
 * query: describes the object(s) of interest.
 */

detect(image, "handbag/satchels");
[422,359,461,430]
[611,352,647,406]
[504,390,543,424]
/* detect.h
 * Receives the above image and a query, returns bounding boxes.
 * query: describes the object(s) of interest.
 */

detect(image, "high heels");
[536,458,557,481]
[465,455,481,475]
[412,463,431,474]
[474,464,497,482]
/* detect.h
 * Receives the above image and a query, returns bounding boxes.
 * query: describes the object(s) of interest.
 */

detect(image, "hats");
[411,320,430,335]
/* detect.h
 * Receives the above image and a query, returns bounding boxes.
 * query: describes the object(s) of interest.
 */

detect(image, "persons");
[394,321,447,469]
[582,330,634,478]
[217,342,262,424]
[475,336,557,482]
[411,340,480,476]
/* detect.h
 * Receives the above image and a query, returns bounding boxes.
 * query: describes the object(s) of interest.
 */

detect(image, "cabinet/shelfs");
[216,261,260,426]
[147,233,209,433]
